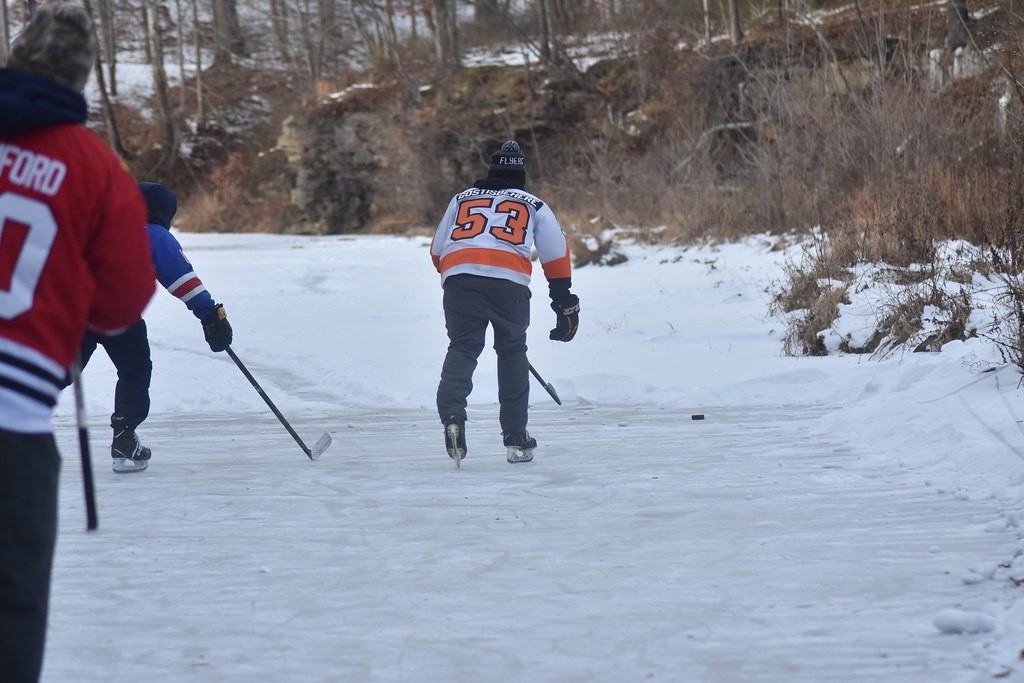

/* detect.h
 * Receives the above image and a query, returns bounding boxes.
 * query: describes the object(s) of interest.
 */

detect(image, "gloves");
[549,294,580,342]
[201,303,233,352]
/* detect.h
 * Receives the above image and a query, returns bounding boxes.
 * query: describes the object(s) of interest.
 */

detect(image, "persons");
[430,141,579,466]
[56,181,232,472]
[0,0,157,683]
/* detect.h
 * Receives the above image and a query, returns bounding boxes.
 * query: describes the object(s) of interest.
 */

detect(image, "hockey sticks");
[529,363,562,405]
[68,347,99,530]
[225,345,332,461]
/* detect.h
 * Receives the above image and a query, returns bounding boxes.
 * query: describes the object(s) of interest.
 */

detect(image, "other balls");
[692,414,704,420]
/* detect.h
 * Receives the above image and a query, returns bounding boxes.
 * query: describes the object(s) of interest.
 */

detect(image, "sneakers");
[108,413,151,473]
[444,414,467,469]
[501,429,537,463]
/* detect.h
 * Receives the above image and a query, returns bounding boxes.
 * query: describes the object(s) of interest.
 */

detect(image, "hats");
[488,141,526,185]
[8,3,94,91]
